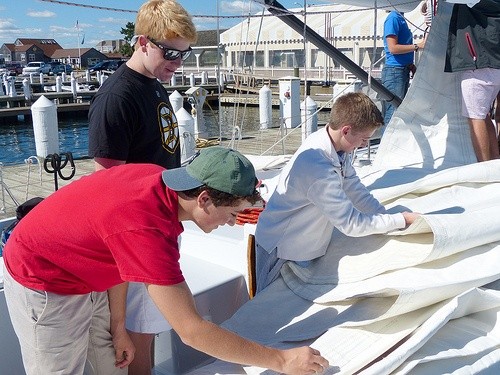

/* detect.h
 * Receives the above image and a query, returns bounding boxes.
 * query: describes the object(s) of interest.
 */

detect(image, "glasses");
[146,35,192,62]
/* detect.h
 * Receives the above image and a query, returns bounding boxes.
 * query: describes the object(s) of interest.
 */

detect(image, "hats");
[161,144,256,197]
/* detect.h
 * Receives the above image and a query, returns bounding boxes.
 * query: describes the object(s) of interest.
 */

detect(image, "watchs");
[414,44,419,52]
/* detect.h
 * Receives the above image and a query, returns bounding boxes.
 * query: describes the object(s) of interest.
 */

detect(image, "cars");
[21,61,51,76]
[87,59,127,75]
[0,61,24,76]
[38,61,73,76]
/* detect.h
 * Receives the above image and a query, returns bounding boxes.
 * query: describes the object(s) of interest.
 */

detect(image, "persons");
[254,91,421,295]
[88,0,198,375]
[381,10,426,136]
[2,146,329,375]
[444,0,500,163]
